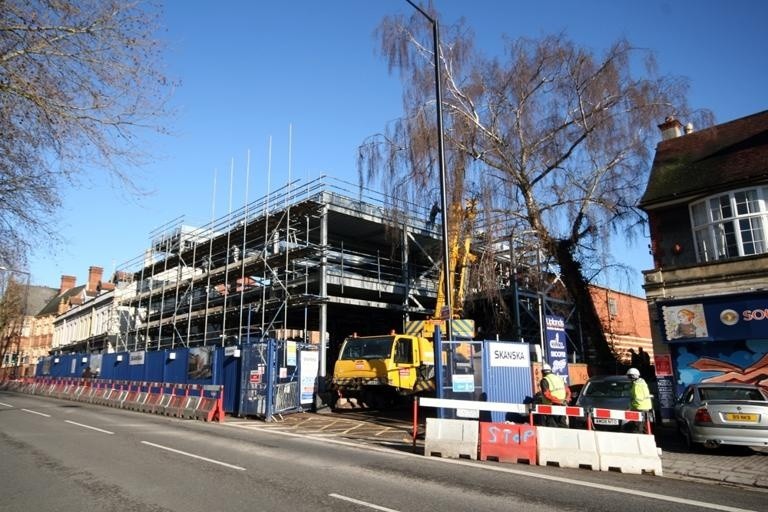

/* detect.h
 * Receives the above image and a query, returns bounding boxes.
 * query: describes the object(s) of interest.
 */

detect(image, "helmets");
[626,368,640,378]
[541,364,551,372]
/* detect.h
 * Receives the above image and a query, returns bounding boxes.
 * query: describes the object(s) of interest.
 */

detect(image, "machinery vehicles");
[334,141,480,403]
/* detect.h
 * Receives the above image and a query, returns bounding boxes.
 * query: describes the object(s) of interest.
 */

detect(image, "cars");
[672,380,768,450]
[573,372,660,432]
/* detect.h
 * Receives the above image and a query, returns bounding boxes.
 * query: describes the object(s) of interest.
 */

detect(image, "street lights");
[0,266,32,379]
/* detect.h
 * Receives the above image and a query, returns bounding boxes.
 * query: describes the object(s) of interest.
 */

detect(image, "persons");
[429,201,442,224]
[81,367,99,387]
[539,363,571,427]
[626,368,652,433]
